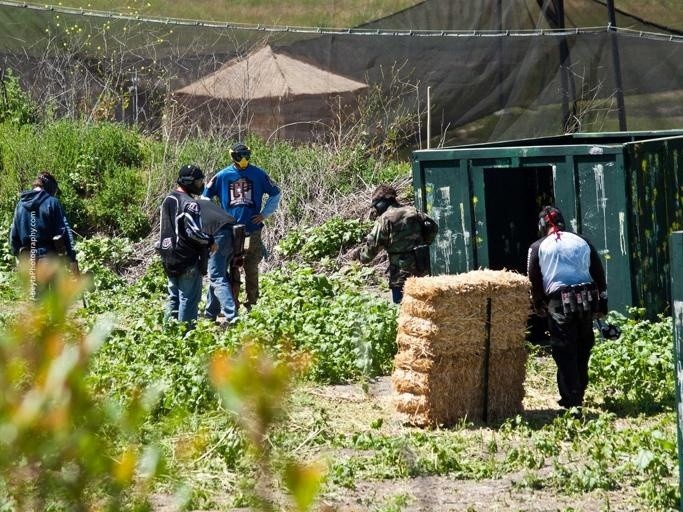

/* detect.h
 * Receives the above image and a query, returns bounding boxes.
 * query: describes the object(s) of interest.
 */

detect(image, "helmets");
[176,164,206,196]
[228,142,251,163]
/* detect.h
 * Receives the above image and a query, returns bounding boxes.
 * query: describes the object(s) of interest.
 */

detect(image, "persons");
[6,171,81,331]
[199,143,279,311]
[346,183,437,308]
[192,197,238,326]
[526,202,609,408]
[151,163,220,335]
